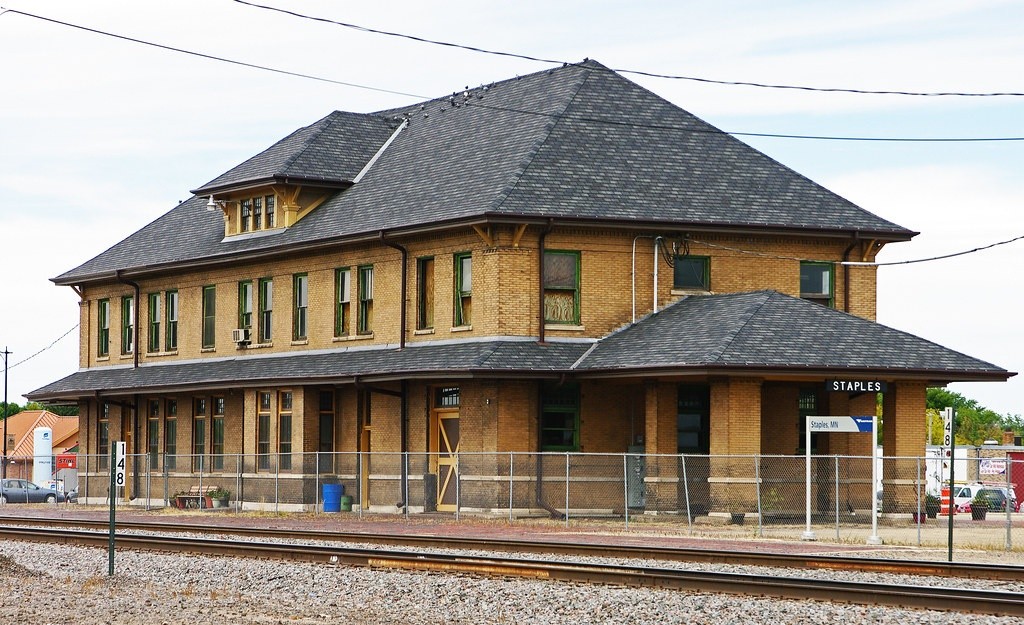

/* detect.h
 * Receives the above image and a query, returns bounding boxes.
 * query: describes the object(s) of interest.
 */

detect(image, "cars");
[65,485,79,503]
[0,478,65,504]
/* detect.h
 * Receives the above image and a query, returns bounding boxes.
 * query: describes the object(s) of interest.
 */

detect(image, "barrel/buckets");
[341,496,352,510]
[323,483,341,512]
[731,513,745,525]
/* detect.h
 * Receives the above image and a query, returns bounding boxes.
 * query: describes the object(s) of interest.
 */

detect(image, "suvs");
[974,484,1018,514]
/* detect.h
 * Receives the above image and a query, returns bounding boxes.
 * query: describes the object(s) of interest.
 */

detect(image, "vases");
[732,513,751,526]
[913,513,926,523]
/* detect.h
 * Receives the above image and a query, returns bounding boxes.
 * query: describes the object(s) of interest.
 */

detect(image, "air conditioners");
[232,329,252,343]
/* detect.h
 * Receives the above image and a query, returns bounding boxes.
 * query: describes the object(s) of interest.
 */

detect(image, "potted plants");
[204,489,231,508]
[169,490,186,508]
[969,493,992,520]
[925,494,940,518]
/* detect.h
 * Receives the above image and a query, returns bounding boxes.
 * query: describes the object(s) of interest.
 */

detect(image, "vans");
[948,483,979,513]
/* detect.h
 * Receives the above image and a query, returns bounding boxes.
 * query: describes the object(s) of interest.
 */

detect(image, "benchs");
[177,485,220,508]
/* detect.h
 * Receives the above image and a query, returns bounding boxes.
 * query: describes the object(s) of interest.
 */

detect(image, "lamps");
[207,195,241,212]
